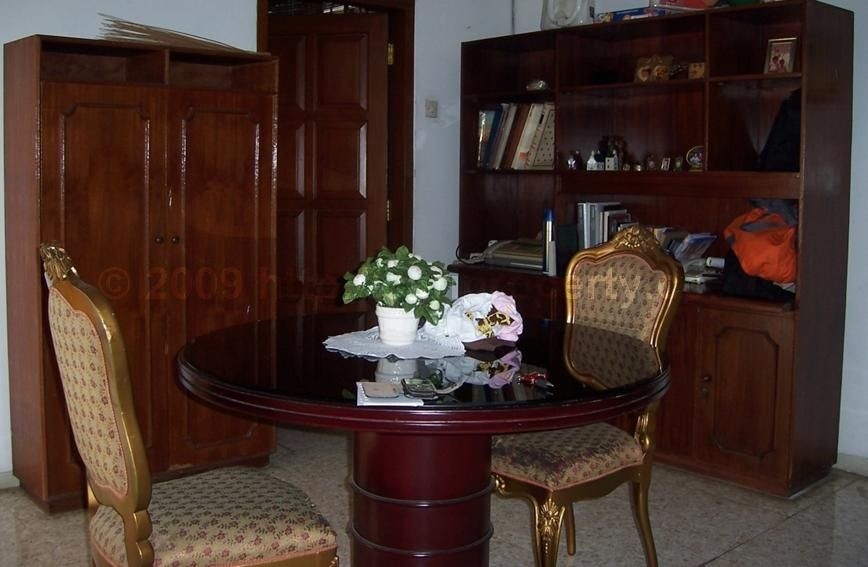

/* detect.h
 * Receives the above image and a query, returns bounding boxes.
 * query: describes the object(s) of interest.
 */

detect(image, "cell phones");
[400,376,437,398]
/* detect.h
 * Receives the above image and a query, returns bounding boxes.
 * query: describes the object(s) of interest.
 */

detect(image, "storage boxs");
[594,6,666,21]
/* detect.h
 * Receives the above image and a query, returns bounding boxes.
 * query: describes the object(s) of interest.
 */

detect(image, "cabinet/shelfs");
[4,35,278,515]
[459,0,854,497]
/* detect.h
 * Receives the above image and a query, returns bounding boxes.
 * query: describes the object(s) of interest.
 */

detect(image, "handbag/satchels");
[724,206,796,283]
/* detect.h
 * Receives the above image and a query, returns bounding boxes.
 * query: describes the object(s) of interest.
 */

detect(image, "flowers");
[344,245,456,325]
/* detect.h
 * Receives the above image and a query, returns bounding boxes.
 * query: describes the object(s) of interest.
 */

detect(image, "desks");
[175,312,672,567]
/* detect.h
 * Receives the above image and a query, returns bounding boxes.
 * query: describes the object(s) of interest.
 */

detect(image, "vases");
[375,359,418,384]
[375,304,421,345]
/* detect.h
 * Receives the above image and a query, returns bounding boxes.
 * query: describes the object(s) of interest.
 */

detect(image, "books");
[476,100,557,172]
[575,202,720,284]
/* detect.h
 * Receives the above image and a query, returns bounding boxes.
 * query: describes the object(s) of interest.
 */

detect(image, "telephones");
[483,238,543,264]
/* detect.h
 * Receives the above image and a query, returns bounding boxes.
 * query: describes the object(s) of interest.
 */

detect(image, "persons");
[768,56,787,72]
[690,152,704,163]
[663,160,668,169]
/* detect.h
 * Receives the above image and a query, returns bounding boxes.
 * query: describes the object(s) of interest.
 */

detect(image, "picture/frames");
[764,38,796,73]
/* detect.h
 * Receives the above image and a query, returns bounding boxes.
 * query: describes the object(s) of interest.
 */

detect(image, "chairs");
[490,226,685,567]
[38,243,339,567]
